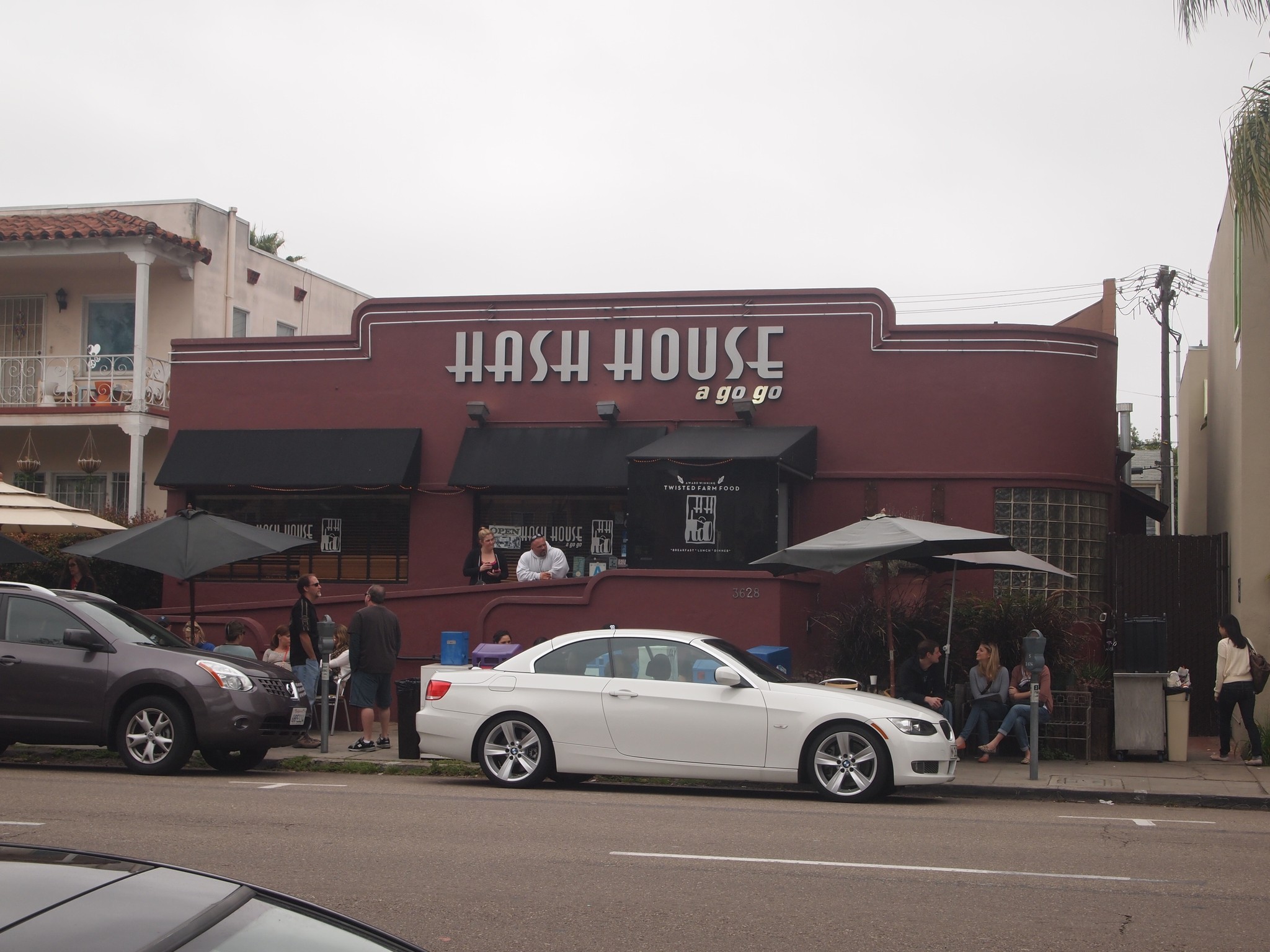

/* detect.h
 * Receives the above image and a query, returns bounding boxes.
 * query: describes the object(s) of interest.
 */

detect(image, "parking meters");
[1022,628,1047,783]
[316,613,337,754]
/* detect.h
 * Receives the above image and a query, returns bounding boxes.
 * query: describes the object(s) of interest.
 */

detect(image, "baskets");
[77,458,102,473]
[17,460,41,474]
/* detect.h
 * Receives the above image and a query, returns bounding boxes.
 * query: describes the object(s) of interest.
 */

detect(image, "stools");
[96,379,114,407]
[38,382,58,408]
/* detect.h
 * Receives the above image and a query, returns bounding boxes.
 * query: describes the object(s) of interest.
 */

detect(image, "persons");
[263,624,293,672]
[289,573,322,750]
[891,639,951,713]
[463,526,509,586]
[1209,613,1264,767]
[315,624,352,696]
[976,646,1053,765]
[347,584,402,751]
[58,553,97,593]
[182,620,215,651]
[944,640,1010,761]
[516,533,570,582]
[494,630,512,646]
[532,636,548,646]
[212,619,258,660]
[154,614,173,632]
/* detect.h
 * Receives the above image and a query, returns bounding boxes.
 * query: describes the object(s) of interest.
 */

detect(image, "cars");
[414,625,960,802]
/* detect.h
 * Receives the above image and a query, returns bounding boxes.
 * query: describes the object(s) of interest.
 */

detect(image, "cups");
[870,675,877,684]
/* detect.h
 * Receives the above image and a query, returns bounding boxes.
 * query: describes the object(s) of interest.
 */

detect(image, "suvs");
[0,580,310,773]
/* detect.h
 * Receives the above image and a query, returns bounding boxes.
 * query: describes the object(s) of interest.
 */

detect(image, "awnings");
[1121,481,1169,524]
[748,507,1078,709]
[1,479,318,649]
[1117,449,1135,467]
[153,421,819,500]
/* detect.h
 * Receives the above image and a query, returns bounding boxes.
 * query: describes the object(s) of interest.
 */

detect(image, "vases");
[869,675,878,685]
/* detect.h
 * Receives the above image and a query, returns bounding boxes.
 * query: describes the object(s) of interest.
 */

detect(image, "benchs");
[960,679,1093,764]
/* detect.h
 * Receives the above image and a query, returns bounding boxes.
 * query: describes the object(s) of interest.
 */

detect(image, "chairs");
[310,668,352,736]
[645,653,672,682]
[36,367,78,408]
[817,677,866,691]
[118,363,172,407]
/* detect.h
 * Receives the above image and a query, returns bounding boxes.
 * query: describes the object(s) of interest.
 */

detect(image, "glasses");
[240,631,246,635]
[365,593,368,596]
[530,533,543,545]
[306,583,320,587]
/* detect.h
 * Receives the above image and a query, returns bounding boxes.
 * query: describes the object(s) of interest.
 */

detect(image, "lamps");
[597,399,621,425]
[55,287,68,313]
[731,398,756,422]
[464,401,491,423]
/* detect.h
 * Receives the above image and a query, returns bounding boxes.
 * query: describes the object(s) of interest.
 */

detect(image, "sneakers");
[293,734,321,748]
[375,733,391,749]
[348,736,376,751]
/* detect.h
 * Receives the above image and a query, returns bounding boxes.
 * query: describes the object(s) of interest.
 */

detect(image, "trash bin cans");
[1162,684,1193,762]
[395,677,421,759]
[1113,668,1170,762]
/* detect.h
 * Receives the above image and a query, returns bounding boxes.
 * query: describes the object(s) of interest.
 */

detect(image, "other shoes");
[1244,760,1262,766]
[978,745,996,753]
[978,754,990,762]
[1021,758,1030,764]
[957,741,966,749]
[1210,754,1230,761]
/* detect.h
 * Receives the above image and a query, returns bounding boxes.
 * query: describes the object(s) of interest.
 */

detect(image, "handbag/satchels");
[1243,636,1270,695]
[1007,679,1044,708]
[475,572,486,585]
